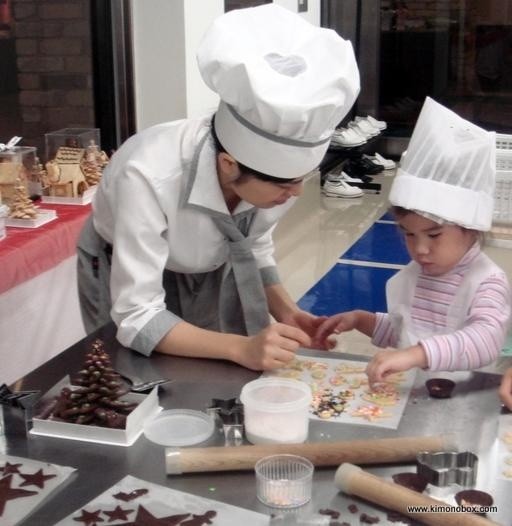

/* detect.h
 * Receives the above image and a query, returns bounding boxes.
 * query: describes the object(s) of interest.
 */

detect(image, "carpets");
[339,221,412,264]
[380,210,399,221]
[296,262,401,317]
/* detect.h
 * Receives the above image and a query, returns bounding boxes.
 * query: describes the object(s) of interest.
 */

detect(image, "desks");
[0,200,96,388]
[0,317,512,526]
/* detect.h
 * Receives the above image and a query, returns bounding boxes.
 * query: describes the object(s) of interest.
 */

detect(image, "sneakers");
[323,115,396,198]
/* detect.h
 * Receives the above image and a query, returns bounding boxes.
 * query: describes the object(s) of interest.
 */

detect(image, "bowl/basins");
[254,454,314,509]
[239,377,313,445]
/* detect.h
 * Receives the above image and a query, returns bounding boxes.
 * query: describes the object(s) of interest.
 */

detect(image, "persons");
[498,368,512,412]
[314,205,511,387]
[76,113,361,371]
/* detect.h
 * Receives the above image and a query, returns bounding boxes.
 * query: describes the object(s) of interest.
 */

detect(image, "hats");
[196,3,361,179]
[390,96,497,231]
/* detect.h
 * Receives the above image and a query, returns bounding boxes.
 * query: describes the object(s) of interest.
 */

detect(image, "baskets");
[494,134,512,225]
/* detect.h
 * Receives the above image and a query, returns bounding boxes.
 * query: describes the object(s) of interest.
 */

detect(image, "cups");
[455,490,494,515]
[426,377,455,398]
[393,473,429,493]
